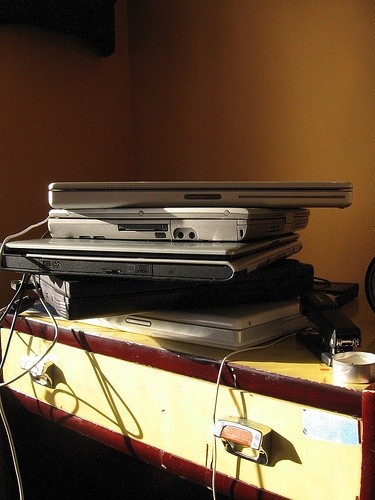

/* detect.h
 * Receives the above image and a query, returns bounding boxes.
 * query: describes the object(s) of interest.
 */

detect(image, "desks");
[0,294,375,500]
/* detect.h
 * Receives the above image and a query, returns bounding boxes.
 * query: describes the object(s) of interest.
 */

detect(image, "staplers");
[297,292,361,366]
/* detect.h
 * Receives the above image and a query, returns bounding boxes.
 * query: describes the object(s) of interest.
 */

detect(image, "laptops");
[0,181,352,352]
